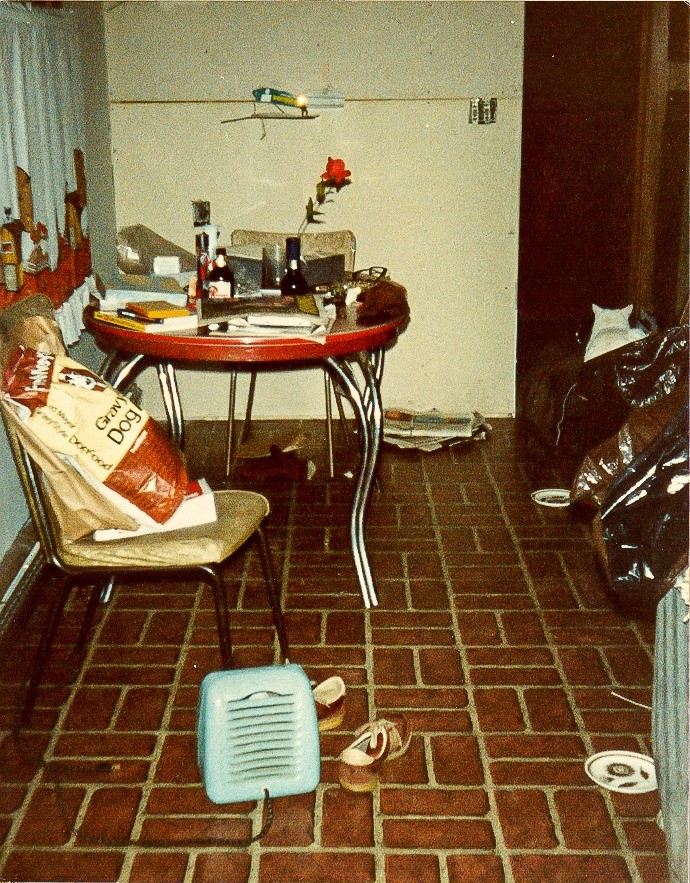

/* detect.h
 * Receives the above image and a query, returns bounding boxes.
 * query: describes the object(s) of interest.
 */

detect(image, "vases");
[279,237,308,296]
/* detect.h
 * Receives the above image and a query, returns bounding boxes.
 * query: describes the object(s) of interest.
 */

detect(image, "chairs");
[0,294,290,733]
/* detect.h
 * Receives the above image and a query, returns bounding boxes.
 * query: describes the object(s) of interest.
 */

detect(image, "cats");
[581,298,660,363]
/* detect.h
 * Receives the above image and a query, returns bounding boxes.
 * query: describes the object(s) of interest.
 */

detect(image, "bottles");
[208,248,234,299]
[281,238,307,295]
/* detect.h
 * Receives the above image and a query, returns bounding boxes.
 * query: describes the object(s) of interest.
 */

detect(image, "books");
[383,407,487,452]
[93,273,314,337]
[93,478,218,542]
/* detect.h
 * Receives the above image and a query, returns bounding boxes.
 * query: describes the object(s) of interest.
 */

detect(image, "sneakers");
[316,674,349,728]
[336,712,412,792]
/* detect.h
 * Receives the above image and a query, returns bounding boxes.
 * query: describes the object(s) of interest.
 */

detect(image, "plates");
[585,750,658,794]
[531,489,571,507]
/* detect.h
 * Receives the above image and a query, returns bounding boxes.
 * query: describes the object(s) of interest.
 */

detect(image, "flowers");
[298,156,355,236]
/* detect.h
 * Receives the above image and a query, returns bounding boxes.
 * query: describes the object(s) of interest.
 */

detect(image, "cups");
[260,246,307,290]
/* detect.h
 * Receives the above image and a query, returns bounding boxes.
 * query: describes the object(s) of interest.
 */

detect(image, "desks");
[80,279,412,608]
